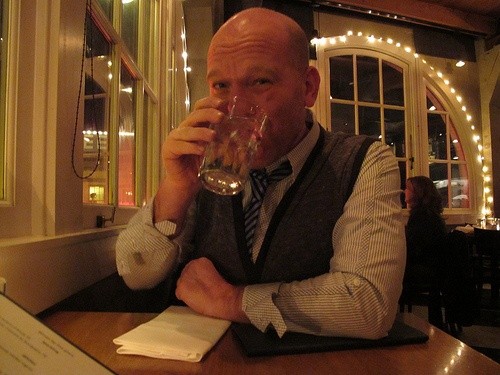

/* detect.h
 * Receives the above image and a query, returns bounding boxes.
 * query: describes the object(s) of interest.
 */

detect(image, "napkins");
[113,306,232,362]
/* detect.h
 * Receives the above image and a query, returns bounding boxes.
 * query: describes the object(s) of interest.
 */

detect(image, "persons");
[399,176,449,304]
[114,8,407,340]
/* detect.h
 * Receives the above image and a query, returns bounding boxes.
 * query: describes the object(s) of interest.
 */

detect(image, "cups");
[199,98,267,194]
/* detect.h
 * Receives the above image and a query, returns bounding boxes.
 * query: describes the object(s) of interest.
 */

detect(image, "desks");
[40,311,500,375]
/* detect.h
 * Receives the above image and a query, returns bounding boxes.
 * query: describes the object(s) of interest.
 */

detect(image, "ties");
[240,160,292,258]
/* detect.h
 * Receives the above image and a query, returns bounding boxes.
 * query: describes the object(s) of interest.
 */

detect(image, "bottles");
[477,217,500,231]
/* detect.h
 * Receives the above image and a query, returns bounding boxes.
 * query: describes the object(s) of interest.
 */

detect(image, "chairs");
[470,226,500,306]
